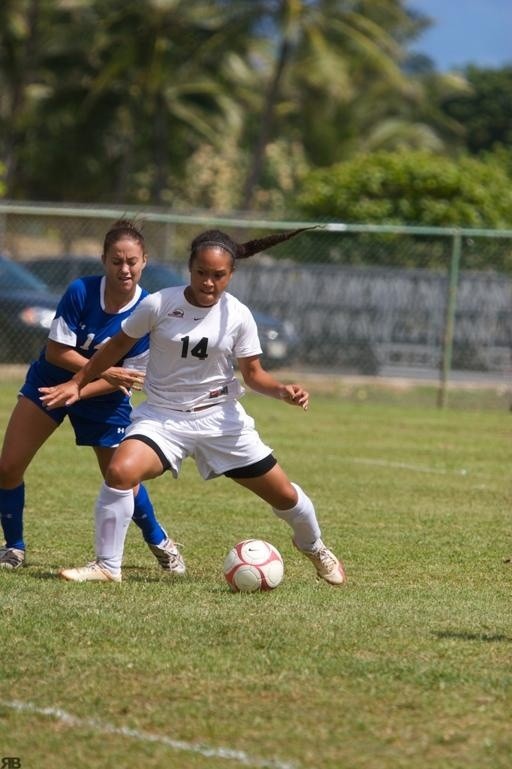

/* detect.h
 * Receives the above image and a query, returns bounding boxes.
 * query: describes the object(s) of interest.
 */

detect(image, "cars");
[1,256,63,343]
[23,257,295,370]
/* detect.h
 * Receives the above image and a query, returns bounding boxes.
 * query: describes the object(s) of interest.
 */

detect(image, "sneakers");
[145,524,184,572]
[0,546,26,571]
[293,535,345,584]
[60,561,123,584]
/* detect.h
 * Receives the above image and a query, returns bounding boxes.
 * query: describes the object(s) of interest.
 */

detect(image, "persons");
[0,219,188,576]
[33,227,348,586]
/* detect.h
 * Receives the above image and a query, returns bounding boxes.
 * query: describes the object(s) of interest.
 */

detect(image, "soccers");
[223,539,284,592]
